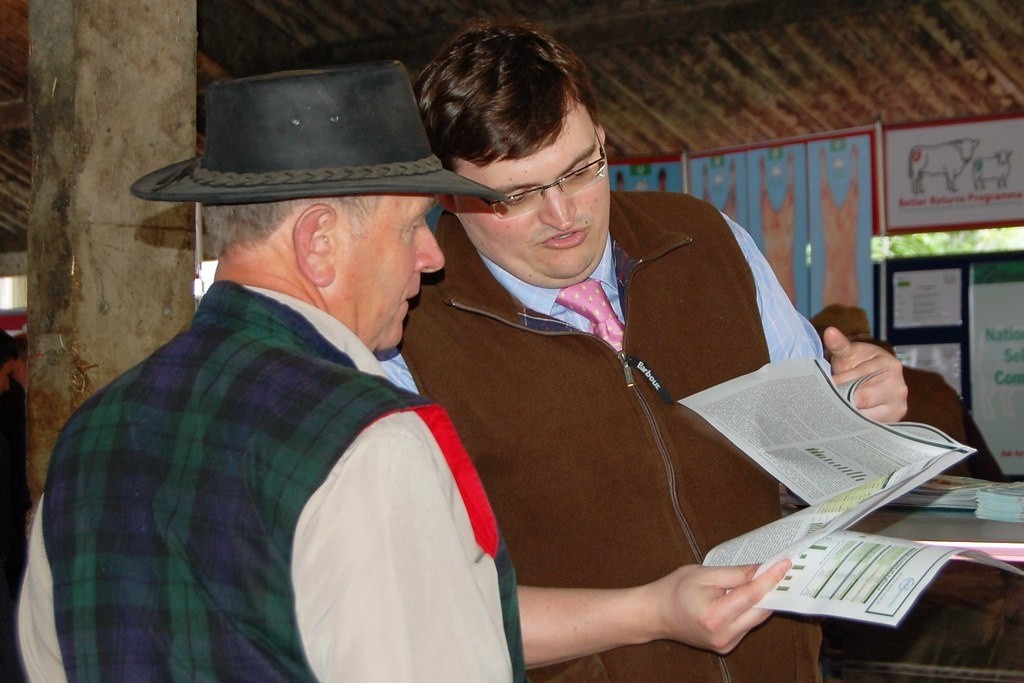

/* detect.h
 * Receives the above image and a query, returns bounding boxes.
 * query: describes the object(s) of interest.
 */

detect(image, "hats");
[809,302,870,339]
[129,58,508,205]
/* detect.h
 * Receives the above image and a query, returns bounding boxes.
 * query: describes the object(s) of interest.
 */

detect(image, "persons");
[809,304,1007,483]
[394,25,907,683]
[0,59,532,683]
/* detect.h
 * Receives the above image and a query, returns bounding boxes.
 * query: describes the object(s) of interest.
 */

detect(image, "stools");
[818,559,1024,683]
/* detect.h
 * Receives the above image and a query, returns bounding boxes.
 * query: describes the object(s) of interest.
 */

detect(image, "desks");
[779,481,1024,563]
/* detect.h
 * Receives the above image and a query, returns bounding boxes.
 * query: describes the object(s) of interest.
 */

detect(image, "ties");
[555,278,626,352]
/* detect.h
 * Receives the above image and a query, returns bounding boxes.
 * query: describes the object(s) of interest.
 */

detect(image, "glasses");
[478,125,607,219]
